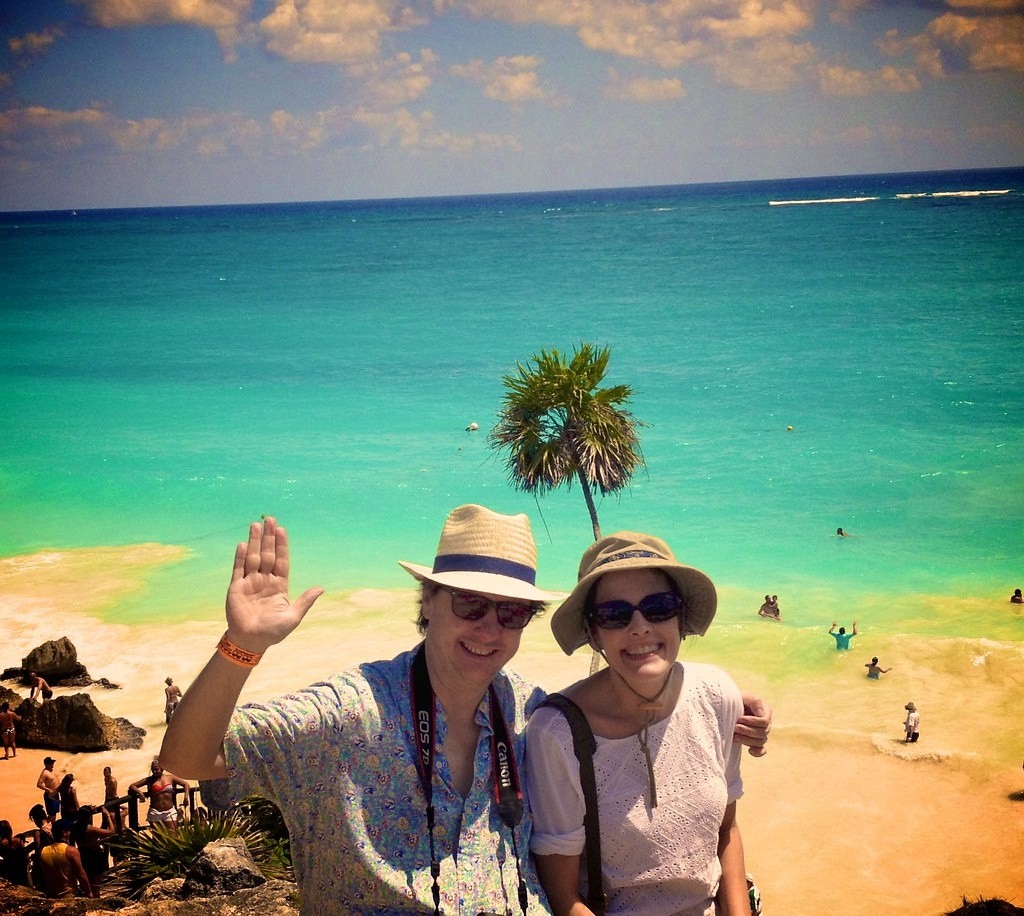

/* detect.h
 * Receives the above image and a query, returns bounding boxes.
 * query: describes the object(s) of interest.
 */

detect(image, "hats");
[398,504,571,602]
[44,757,56,764]
[905,702,916,710]
[551,531,717,656]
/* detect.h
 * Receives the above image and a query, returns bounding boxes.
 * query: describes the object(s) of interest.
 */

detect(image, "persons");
[526,530,750,915]
[158,503,772,916]
[1,671,182,900]
[759,528,1024,743]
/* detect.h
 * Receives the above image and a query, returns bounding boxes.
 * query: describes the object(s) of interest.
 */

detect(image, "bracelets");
[218,630,264,669]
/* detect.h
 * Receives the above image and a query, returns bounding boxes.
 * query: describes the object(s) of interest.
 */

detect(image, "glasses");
[584,591,684,630]
[439,586,538,629]
